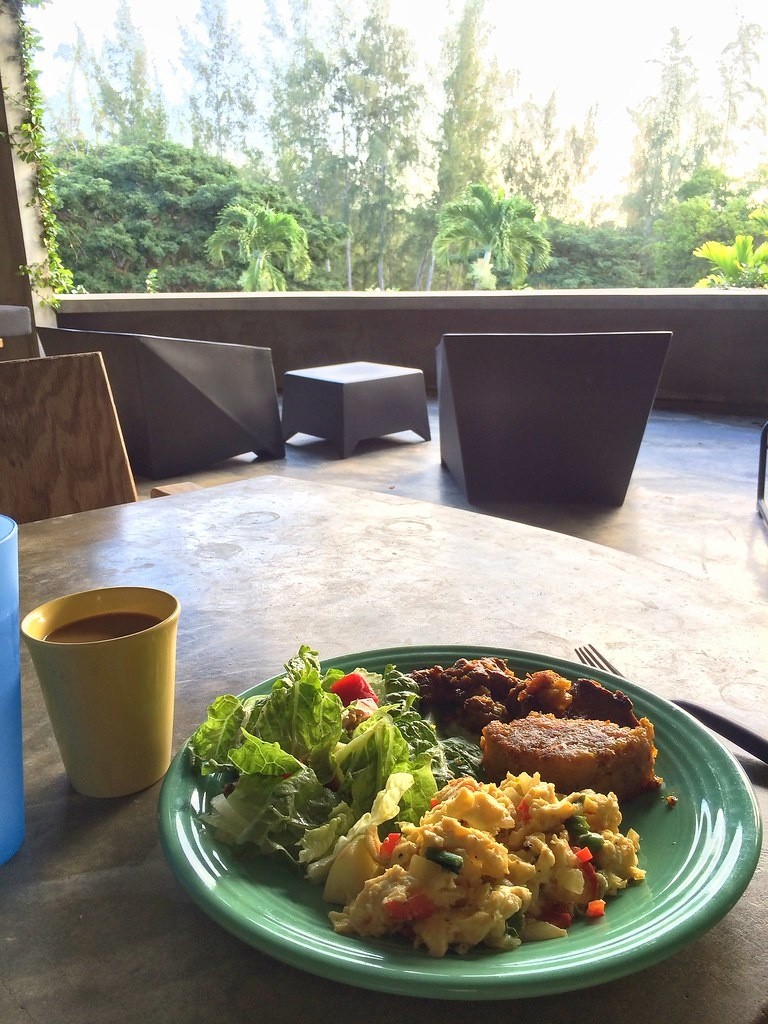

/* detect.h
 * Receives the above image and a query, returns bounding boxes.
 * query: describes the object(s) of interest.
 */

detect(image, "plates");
[156,644,762,1003]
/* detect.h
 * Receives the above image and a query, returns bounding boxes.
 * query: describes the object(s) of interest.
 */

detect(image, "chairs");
[1,350,142,524]
[436,329,679,514]
[37,324,279,487]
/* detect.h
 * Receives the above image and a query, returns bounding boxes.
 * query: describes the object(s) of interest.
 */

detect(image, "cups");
[21,587,180,799]
[0,513,27,868]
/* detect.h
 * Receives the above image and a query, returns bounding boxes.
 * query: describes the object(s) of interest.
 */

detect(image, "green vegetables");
[189,646,483,902]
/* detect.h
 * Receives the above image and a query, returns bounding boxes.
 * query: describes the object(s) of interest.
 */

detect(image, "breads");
[480,710,662,803]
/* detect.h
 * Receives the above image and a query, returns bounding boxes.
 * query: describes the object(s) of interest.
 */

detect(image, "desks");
[2,475,768,1023]
[280,361,427,457]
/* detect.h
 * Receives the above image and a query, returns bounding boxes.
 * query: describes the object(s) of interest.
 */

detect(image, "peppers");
[379,794,605,928]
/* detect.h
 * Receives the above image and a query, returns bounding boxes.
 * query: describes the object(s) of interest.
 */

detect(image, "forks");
[574,645,768,764]
